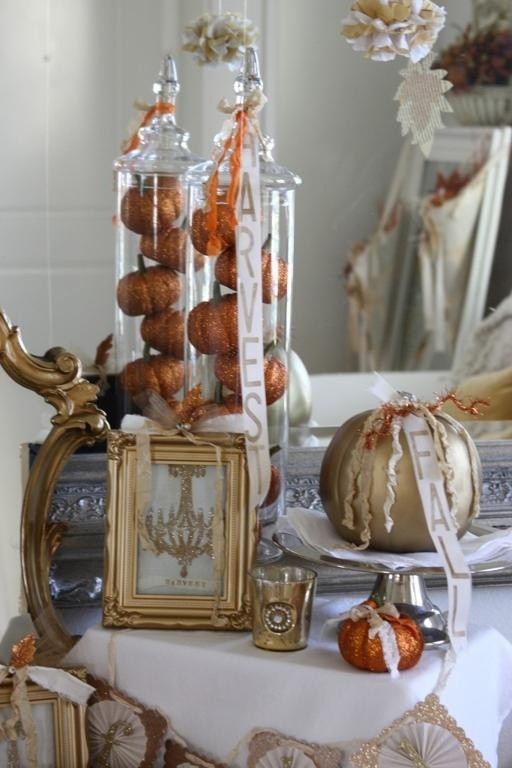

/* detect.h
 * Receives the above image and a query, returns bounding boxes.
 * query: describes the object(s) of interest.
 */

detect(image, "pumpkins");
[337,599,424,673]
[117,176,287,430]
[258,445,280,509]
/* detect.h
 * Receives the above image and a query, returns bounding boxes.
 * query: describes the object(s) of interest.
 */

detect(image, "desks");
[58,585,511,768]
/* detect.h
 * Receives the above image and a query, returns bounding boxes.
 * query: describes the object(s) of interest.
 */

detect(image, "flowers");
[182,2,256,67]
[437,10,512,97]
[339,1,452,159]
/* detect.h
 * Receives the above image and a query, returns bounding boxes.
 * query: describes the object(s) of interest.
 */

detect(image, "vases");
[445,84,512,124]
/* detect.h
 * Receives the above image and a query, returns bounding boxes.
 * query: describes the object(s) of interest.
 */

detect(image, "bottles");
[113,54,208,432]
[185,48,303,560]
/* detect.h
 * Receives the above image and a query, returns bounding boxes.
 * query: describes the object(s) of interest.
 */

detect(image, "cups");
[248,564,316,651]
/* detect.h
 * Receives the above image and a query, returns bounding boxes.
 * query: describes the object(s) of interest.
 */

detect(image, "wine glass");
[276,523,512,648]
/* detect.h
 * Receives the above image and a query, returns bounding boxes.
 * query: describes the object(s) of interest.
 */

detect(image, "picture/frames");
[376,123,512,372]
[102,429,261,632]
[0,664,91,768]
